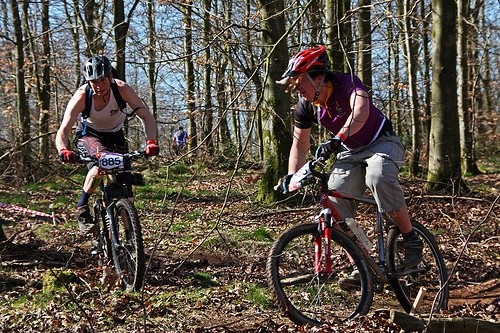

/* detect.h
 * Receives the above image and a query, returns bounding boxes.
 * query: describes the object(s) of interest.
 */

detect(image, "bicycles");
[266,148,449,325]
[56,147,160,293]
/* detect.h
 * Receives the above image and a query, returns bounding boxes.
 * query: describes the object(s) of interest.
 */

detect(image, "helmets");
[276,44,331,96]
[82,54,117,89]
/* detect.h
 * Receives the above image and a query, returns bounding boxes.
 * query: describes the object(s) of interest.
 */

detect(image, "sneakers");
[73,203,97,233]
[402,234,424,269]
[338,267,384,295]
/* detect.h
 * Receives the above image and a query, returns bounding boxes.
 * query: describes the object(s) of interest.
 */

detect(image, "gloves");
[145,139,160,157]
[315,137,343,161]
[281,173,293,195]
[58,149,79,164]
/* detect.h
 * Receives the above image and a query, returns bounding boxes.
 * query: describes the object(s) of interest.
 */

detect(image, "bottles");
[345,217,375,251]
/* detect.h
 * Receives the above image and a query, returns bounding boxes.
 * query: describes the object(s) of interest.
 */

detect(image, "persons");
[274,45,424,290]
[173,126,189,145]
[55,55,160,254]
